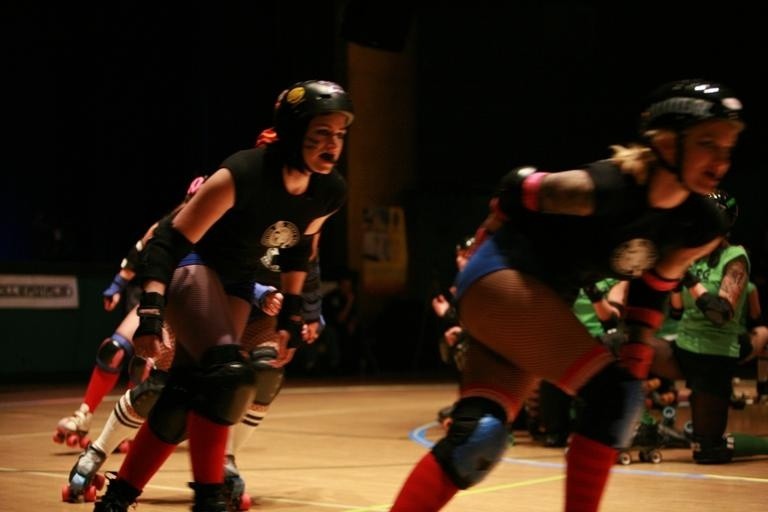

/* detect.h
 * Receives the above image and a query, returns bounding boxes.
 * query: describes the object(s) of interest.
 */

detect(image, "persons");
[432,234,480,417]
[56,128,325,508]
[389,78,744,511]
[96,81,357,511]
[529,190,767,462]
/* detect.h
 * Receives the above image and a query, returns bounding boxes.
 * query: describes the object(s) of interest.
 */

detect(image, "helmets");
[255,80,356,149]
[187,175,209,195]
[644,83,745,185]
[455,236,473,260]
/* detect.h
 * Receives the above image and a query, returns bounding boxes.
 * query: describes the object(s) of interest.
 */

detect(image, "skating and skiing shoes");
[437,403,457,430]
[62,440,144,512]
[188,454,251,512]
[618,379,696,466]
[52,402,92,448]
